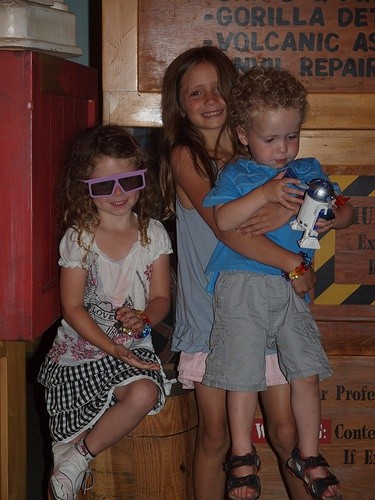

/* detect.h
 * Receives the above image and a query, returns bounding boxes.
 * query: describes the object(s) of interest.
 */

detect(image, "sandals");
[286,445,344,500]
[225,443,263,500]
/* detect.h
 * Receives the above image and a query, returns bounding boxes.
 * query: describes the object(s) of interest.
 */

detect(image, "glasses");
[80,168,147,198]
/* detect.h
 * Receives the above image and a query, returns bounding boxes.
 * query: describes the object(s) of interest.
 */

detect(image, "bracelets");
[114,308,152,340]
[280,251,312,281]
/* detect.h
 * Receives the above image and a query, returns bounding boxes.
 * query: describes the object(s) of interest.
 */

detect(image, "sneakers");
[48,442,91,499]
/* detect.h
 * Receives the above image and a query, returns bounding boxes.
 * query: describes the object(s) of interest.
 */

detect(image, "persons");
[35,125,174,500]
[160,46,314,500]
[201,65,354,500]
